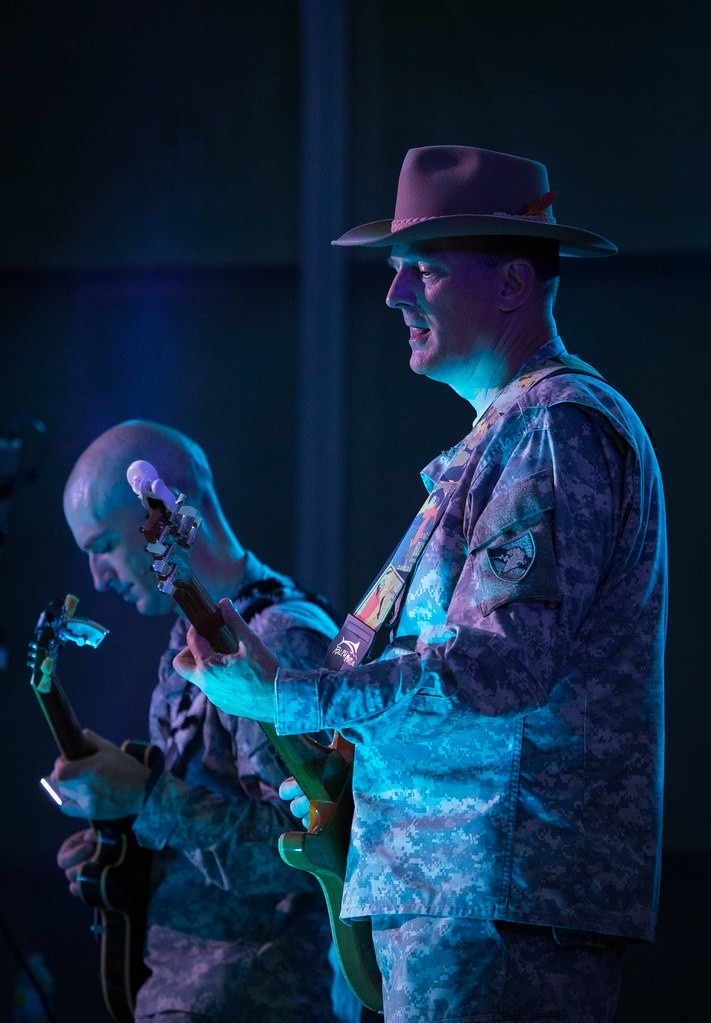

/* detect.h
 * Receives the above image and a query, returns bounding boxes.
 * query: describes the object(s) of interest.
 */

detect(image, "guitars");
[24,590,166,1023]
[122,454,385,1015]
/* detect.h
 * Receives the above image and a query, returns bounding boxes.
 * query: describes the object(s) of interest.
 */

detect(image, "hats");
[330,146,619,257]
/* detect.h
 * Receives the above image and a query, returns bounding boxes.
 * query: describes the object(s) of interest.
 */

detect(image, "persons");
[41,420,339,1023]
[169,143,670,1023]
